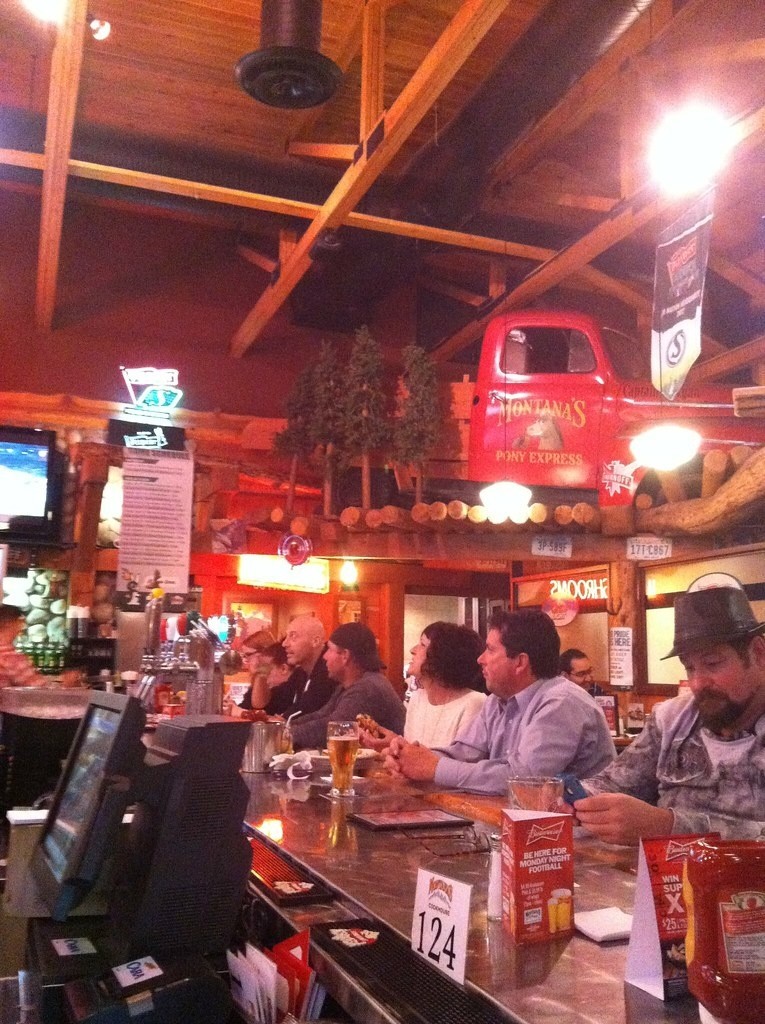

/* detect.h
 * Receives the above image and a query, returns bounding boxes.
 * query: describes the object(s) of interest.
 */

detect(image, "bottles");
[175,634,191,663]
[87,643,113,675]
[114,670,121,685]
[158,640,174,666]
[682,840,765,1024]
[126,680,135,694]
[485,833,502,920]
[69,642,83,669]
[548,889,572,933]
[15,642,65,675]
[100,670,110,682]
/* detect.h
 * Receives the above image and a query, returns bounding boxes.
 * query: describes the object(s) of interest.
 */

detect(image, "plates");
[307,749,378,768]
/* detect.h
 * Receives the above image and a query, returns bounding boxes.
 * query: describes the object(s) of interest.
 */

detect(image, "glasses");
[240,650,260,658]
[569,669,592,677]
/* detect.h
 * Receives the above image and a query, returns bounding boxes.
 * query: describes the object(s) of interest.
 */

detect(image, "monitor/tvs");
[0,425,58,536]
[25,691,147,919]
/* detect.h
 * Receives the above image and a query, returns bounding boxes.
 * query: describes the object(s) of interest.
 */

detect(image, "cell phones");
[554,774,588,805]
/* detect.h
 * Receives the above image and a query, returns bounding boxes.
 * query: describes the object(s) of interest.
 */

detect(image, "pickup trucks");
[330,305,765,537]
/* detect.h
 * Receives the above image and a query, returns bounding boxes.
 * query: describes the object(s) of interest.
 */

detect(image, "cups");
[327,721,360,797]
[507,777,563,814]
[243,722,281,773]
[281,727,294,754]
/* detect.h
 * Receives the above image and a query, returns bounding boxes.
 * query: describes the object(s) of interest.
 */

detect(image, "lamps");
[231,0,342,109]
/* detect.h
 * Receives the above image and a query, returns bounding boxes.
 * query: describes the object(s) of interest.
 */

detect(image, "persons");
[288,623,407,747]
[238,631,302,714]
[225,615,338,721]
[382,610,618,793]
[548,586,764,840]
[361,621,488,751]
[559,648,606,697]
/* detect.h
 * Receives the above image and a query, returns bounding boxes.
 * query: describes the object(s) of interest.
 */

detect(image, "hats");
[660,586,765,661]
[327,622,388,672]
